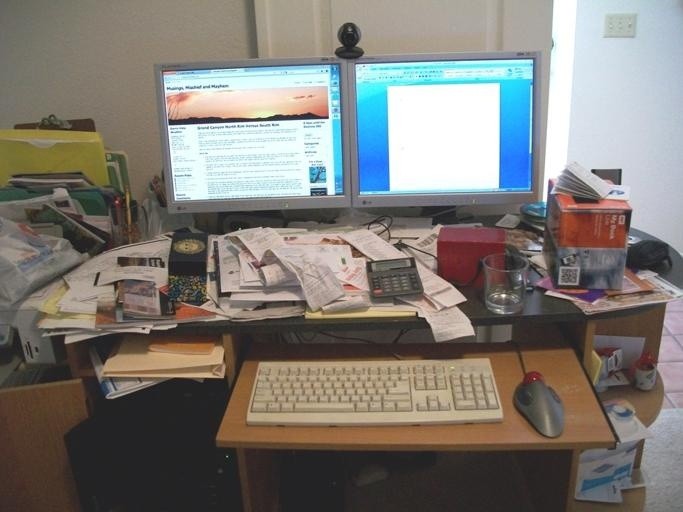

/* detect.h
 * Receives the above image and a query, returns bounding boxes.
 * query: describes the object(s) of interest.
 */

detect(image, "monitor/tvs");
[348,48,555,228]
[152,56,352,228]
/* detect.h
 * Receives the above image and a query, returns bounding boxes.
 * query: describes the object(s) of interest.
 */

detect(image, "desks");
[63,267,669,512]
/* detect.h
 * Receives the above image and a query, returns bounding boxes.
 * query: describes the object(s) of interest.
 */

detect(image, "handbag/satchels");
[628,240,668,270]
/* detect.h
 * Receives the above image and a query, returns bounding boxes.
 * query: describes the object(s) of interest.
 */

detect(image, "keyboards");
[245,356,505,426]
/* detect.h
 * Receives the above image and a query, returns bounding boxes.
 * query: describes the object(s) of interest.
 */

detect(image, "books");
[94,254,216,328]
[7,170,93,192]
[24,202,109,252]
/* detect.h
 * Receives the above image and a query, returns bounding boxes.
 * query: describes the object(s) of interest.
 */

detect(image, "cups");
[633,365,657,391]
[108,205,138,247]
[482,253,530,315]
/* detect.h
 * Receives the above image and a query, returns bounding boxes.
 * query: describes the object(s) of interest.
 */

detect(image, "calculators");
[365,256,423,302]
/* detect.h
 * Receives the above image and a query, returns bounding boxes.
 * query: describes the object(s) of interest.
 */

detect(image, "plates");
[520,202,547,224]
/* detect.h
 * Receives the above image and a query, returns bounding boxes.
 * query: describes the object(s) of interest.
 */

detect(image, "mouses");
[513,370,567,438]
